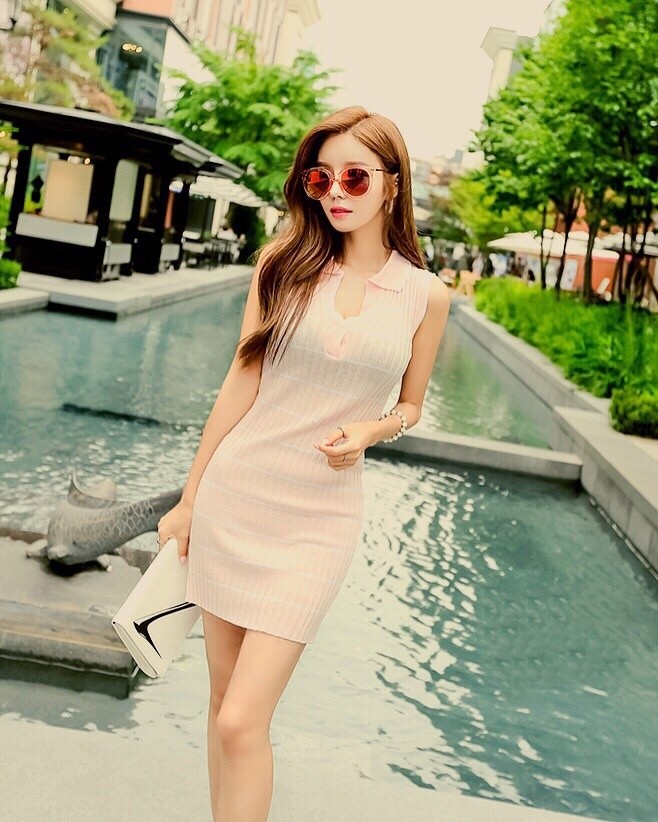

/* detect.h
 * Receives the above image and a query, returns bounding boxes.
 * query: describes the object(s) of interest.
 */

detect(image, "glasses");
[299,166,391,200]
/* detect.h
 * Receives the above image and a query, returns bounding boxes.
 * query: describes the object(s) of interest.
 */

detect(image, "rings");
[343,454,348,463]
[338,427,345,437]
[157,538,164,550]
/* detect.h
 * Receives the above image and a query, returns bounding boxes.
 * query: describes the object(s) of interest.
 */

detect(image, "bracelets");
[379,410,407,443]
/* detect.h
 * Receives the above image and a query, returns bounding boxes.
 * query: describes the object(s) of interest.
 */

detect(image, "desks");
[158,244,180,273]
[101,243,132,281]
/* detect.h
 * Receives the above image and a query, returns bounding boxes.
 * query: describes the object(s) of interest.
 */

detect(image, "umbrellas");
[169,175,268,243]
[487,228,615,282]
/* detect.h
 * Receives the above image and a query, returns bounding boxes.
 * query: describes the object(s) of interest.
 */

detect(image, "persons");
[217,225,234,240]
[240,215,261,265]
[84,212,98,224]
[157,107,451,822]
[422,236,536,298]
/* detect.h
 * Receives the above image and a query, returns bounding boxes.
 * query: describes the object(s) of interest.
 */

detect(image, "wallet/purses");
[111,537,203,679]
